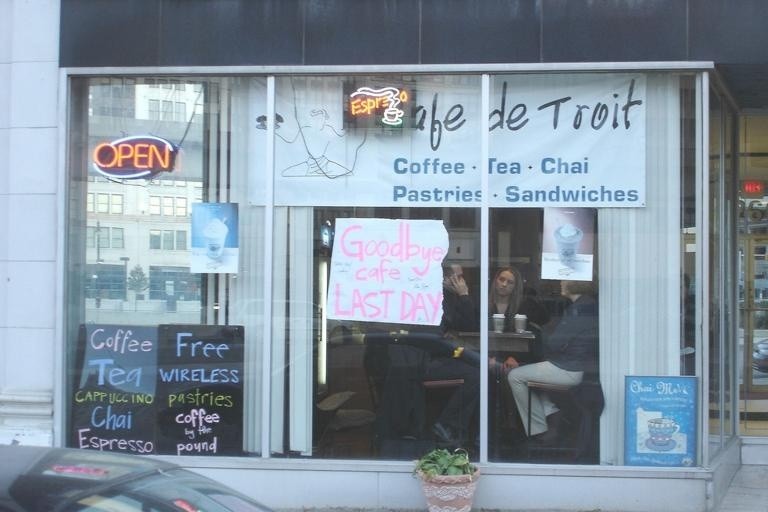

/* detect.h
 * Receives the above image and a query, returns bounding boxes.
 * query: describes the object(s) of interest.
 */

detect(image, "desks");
[457,330,535,353]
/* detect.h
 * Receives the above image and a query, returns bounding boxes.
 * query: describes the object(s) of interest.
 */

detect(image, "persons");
[680,274,696,350]
[489,269,528,418]
[407,258,481,442]
[507,280,599,458]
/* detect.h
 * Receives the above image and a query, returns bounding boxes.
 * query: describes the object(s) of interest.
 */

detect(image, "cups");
[514,313,528,334]
[203,223,228,259]
[555,225,583,262]
[493,314,506,334]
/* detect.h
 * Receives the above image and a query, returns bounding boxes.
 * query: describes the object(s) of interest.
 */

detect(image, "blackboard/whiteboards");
[155,323,245,457]
[68,322,158,457]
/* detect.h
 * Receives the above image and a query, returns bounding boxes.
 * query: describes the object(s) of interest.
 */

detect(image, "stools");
[422,378,580,460]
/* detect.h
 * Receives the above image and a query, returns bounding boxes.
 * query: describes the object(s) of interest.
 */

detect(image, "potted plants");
[413,447,481,512]
